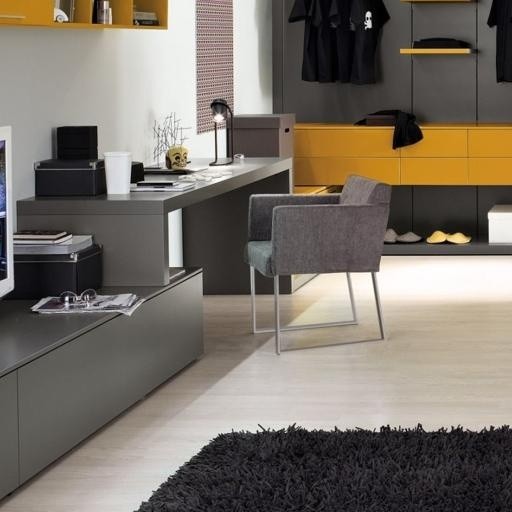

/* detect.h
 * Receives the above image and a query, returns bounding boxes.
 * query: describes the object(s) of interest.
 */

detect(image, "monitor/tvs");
[0,125,15,299]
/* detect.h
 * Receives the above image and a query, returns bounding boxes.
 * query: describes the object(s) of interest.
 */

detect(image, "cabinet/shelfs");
[1,0,169,30]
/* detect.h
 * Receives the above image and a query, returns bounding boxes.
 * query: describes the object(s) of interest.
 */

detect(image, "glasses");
[54,288,97,305]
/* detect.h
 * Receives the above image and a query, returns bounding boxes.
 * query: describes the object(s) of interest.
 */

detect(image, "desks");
[1,155,295,504]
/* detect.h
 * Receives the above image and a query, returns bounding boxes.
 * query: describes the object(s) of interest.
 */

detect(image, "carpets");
[135,420,512,511]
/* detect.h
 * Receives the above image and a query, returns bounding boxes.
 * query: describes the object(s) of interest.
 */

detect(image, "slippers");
[395,231,423,242]
[447,232,473,244]
[384,228,399,243]
[426,230,450,243]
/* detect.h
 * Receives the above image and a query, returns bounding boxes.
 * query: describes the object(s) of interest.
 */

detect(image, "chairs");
[244,173,397,355]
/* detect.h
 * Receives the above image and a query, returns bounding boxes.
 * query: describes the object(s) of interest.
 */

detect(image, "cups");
[103,151,133,195]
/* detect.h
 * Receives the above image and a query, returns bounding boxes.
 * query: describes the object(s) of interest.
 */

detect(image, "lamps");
[209,97,235,167]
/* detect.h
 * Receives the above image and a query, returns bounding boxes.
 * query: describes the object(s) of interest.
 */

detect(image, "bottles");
[97,0,109,24]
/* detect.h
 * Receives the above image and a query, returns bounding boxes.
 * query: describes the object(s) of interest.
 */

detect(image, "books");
[30,292,149,317]
[94,0,113,24]
[13,229,93,255]
[133,12,160,26]
[130,180,195,192]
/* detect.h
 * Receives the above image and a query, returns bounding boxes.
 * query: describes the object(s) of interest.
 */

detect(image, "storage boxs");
[227,113,295,158]
